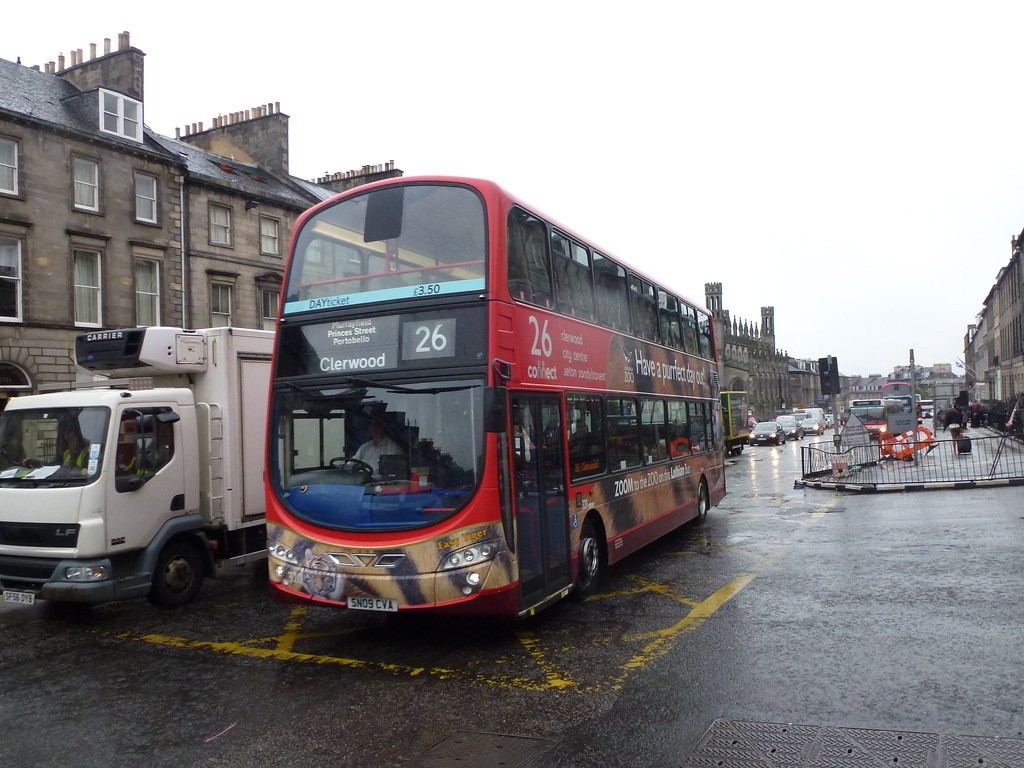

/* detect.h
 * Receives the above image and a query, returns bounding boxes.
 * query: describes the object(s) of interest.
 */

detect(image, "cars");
[748,407,842,447]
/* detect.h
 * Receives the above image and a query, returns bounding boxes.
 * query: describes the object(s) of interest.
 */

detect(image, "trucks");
[720,391,750,459]
[0,325,279,611]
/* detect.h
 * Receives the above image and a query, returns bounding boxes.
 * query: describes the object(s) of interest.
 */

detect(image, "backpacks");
[962,415,968,423]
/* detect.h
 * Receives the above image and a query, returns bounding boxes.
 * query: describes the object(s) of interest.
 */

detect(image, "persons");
[334,413,404,483]
[61,430,89,470]
[944,404,964,455]
[953,401,991,428]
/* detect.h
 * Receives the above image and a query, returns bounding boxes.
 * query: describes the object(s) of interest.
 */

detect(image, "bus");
[848,383,924,439]
[261,174,728,624]
[921,399,934,418]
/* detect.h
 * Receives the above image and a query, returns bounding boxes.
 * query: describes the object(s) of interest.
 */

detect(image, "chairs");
[509,279,705,358]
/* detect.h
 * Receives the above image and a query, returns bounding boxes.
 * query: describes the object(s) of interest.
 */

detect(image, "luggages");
[957,432,971,454]
